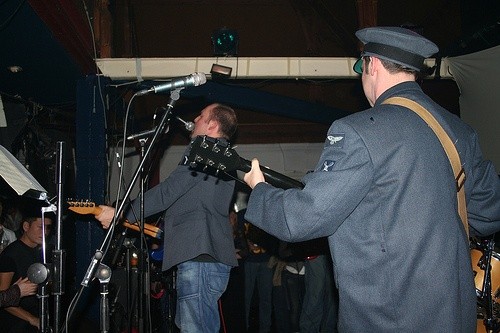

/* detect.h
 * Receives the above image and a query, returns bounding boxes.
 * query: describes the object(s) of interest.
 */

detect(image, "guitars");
[67,196,169,261]
[181,135,304,196]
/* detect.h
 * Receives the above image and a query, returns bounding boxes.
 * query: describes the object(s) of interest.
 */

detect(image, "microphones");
[136,72,207,98]
[162,105,195,131]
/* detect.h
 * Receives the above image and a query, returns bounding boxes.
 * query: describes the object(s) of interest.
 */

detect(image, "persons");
[94,103,239,333]
[0,209,53,333]
[104,198,136,333]
[244,26,500,333]
[297,237,339,332]
[0,272,38,311]
[235,206,280,332]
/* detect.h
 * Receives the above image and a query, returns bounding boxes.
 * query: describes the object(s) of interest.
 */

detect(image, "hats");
[353,26,440,75]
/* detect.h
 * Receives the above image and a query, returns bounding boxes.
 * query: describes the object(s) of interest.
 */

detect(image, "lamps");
[211,24,241,57]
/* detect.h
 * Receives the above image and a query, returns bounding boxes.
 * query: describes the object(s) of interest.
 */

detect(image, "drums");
[473,306,500,333]
[471,243,500,306]
